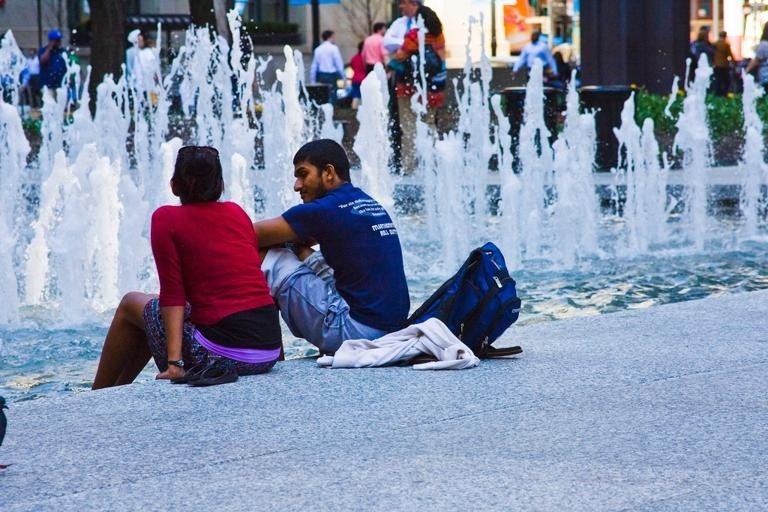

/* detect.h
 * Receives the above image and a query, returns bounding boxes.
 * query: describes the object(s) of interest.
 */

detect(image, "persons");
[693,28,715,71]
[38,30,69,104]
[711,31,739,97]
[308,29,346,106]
[125,28,154,100]
[360,21,389,73]
[26,46,40,94]
[64,44,82,104]
[745,21,768,95]
[251,138,410,356]
[394,6,450,129]
[382,0,443,173]
[91,145,284,394]
[349,40,367,110]
[510,31,557,85]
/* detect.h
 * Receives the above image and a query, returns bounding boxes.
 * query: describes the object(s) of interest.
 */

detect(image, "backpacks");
[396,242,523,362]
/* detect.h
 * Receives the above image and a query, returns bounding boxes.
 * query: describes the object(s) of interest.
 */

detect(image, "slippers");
[170,362,239,386]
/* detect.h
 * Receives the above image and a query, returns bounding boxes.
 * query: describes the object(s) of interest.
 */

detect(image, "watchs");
[167,359,184,368]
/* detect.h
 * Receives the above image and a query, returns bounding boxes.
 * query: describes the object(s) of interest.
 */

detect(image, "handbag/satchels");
[407,44,442,83]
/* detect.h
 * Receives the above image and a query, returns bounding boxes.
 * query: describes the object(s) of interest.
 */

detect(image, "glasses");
[178,146,218,156]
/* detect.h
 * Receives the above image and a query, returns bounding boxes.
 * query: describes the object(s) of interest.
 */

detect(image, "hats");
[48,29,62,39]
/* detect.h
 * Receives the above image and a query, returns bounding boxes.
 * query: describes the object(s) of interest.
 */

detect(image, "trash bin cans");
[578,85,638,171]
[499,87,559,173]
